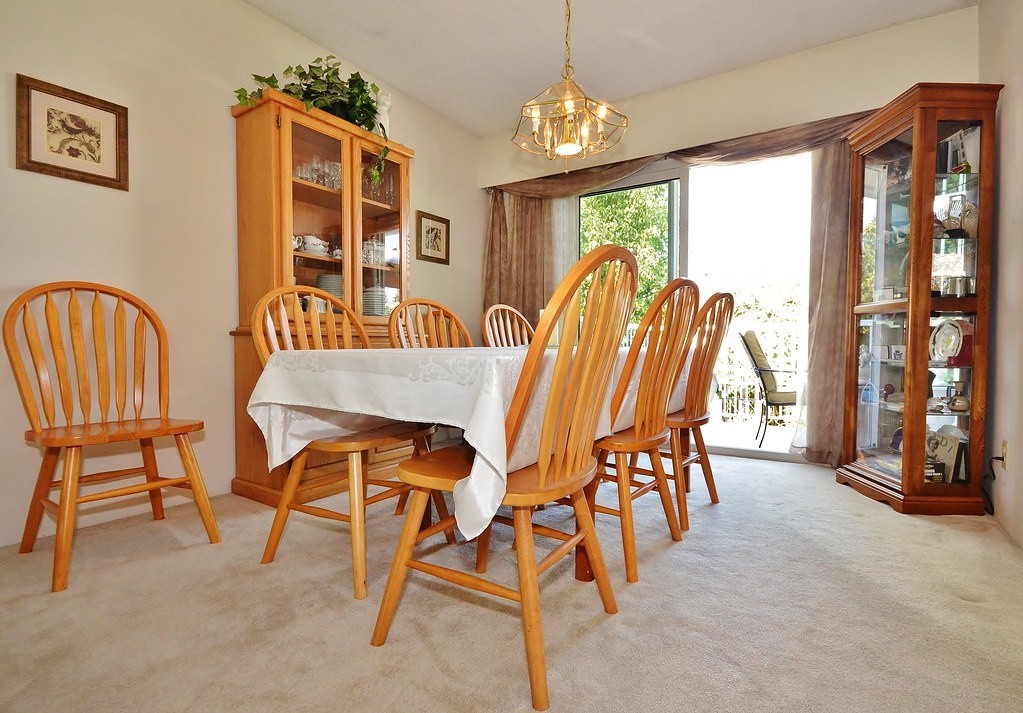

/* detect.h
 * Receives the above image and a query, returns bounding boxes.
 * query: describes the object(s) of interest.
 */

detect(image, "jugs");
[303,295,334,313]
[302,235,330,256]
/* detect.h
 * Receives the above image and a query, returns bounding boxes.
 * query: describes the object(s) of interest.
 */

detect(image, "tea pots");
[292,236,302,251]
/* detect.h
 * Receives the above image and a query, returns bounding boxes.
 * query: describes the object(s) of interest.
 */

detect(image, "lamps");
[510,0,629,175]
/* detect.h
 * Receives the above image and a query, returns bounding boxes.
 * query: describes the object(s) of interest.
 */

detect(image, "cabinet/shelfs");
[229,88,416,508]
[836,83,1005,516]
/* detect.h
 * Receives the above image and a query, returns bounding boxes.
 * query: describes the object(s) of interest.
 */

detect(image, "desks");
[246,344,719,539]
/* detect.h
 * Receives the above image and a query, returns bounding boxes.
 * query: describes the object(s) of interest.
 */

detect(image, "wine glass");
[295,156,396,207]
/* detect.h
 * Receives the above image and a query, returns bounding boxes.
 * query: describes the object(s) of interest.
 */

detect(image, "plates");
[316,274,343,313]
[936,201,979,235]
[363,287,396,316]
[929,319,963,364]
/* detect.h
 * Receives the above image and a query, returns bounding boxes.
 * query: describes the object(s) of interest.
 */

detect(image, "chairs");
[739,330,797,450]
[370,245,638,710]
[251,285,456,601]
[481,302,610,483]
[388,298,546,542]
[511,275,701,584]
[2,281,221,593]
[631,292,735,529]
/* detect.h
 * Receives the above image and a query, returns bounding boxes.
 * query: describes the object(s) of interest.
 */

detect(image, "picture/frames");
[15,72,129,193]
[416,210,450,266]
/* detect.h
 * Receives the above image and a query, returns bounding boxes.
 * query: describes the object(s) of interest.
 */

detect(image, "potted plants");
[230,54,390,186]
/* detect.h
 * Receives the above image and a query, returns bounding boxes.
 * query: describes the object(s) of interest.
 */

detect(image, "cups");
[891,344,906,361]
[940,277,956,298]
[948,194,967,229]
[955,277,971,297]
